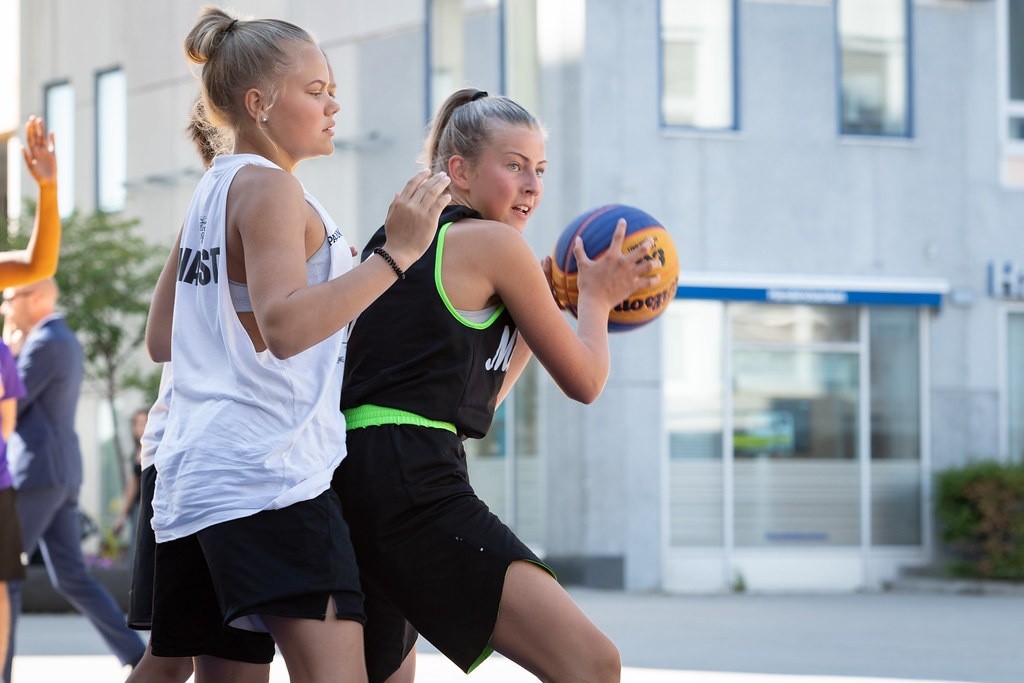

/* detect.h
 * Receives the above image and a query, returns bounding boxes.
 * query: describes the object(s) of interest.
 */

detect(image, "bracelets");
[373,246,406,280]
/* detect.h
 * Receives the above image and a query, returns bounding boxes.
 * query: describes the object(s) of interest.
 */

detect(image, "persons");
[0,4,662,683]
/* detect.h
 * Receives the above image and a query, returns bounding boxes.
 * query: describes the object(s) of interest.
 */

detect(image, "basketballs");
[550,201,682,337]
